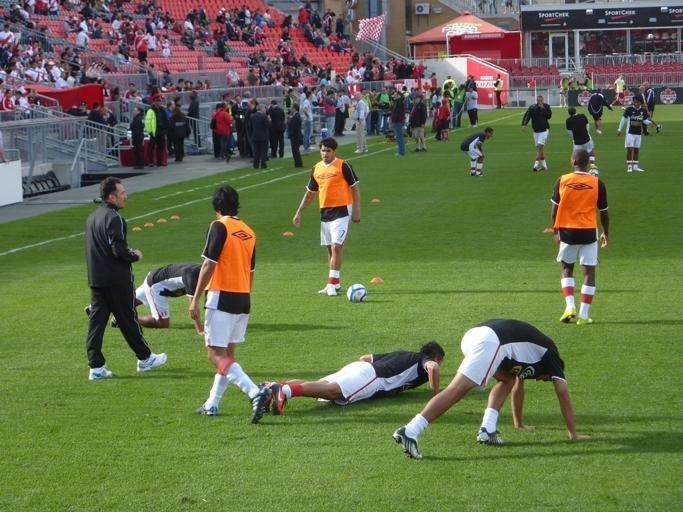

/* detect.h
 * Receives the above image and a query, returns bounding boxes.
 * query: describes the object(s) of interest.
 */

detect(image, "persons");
[83,176,167,380]
[388,316,594,459]
[256,340,448,416]
[548,148,612,327]
[522,96,552,171]
[82,258,207,336]
[559,73,661,177]
[493,74,503,110]
[188,182,273,425]
[292,137,362,297]
[1,0,478,171]
[460,128,493,177]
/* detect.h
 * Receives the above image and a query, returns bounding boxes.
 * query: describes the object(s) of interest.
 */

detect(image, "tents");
[407,14,506,60]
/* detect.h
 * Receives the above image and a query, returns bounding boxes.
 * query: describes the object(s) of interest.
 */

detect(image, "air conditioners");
[415,3,429,15]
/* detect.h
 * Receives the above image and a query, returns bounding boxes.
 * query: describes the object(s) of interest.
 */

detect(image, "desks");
[118,135,168,167]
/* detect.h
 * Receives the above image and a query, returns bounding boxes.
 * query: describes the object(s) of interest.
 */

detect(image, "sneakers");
[392,427,423,460]
[597,129,601,134]
[111,315,119,328]
[560,307,577,323]
[137,352,167,372]
[627,165,632,173]
[327,286,337,296]
[261,165,267,169]
[610,104,613,110]
[148,164,153,167]
[270,382,287,415]
[538,166,547,170]
[473,125,477,128]
[84,303,92,320]
[254,165,259,170]
[471,172,475,176]
[133,166,143,169]
[476,427,503,446]
[421,148,426,151]
[657,125,662,133]
[279,153,283,158]
[89,367,112,380]
[394,153,402,156]
[251,385,272,424]
[355,149,362,153]
[533,166,537,172]
[318,284,340,293]
[576,317,593,326]
[644,132,650,136]
[157,163,167,167]
[363,148,368,152]
[476,173,483,176]
[197,404,218,416]
[589,169,599,175]
[634,165,644,172]
[621,107,625,110]
[415,148,420,152]
[270,154,277,158]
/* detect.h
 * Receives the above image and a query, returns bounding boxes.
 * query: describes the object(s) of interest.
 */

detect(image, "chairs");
[508,59,683,89]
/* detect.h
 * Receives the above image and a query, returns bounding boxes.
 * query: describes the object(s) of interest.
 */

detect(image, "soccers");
[347,283,367,302]
